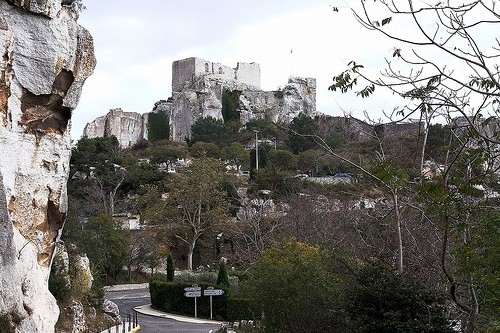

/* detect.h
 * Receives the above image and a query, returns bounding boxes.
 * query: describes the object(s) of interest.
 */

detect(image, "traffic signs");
[184,285,201,298]
[203,286,224,296]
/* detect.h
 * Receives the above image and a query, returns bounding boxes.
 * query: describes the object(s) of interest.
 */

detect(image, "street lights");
[252,128,261,172]
